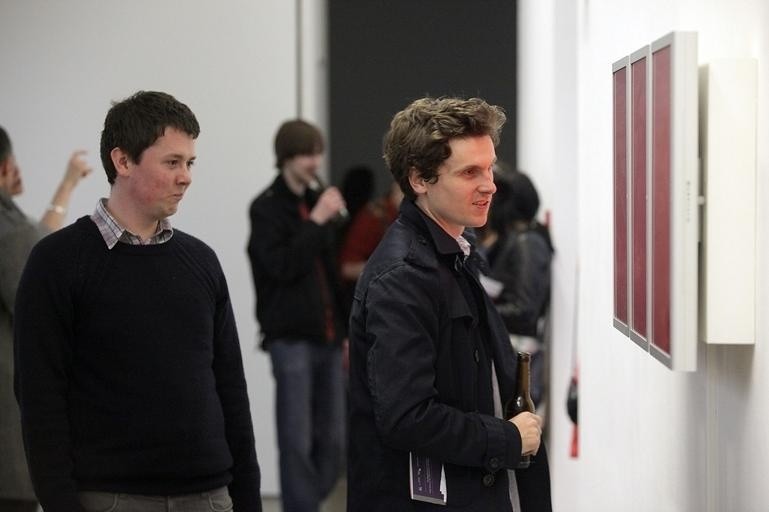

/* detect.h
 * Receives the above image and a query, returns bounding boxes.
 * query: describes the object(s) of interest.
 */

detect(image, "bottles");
[507,350,536,469]
[304,170,351,225]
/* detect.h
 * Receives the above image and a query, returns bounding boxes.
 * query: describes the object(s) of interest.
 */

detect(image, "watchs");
[46,203,70,217]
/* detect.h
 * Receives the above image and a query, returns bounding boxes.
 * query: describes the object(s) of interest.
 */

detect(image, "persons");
[242,114,351,511]
[478,167,556,409]
[1,147,94,512]
[338,174,405,282]
[10,88,266,512]
[1,126,13,183]
[342,89,555,511]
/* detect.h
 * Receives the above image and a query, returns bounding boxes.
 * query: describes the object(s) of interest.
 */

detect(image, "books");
[406,450,448,506]
